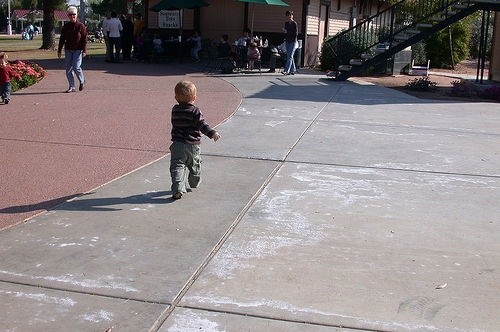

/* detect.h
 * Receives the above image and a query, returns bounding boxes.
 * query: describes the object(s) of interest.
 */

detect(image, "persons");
[57,6,88,93]
[152,30,163,54]
[186,29,204,63]
[175,29,185,64]
[167,79,222,200]
[84,9,146,64]
[21,22,40,40]
[280,9,300,75]
[0,51,22,104]
[212,28,287,74]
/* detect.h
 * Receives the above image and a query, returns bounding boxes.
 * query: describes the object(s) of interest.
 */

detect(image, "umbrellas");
[231,0,290,32]
[148,0,210,35]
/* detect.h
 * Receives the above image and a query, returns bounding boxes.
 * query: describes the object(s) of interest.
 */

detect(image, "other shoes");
[281,71,290,75]
[174,191,182,198]
[66,86,76,93]
[79,80,85,90]
[291,71,294,75]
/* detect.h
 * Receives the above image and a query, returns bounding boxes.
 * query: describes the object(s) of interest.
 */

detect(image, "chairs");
[199,39,210,59]
[212,39,303,73]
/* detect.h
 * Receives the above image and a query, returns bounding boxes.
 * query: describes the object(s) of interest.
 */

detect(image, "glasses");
[68,13,77,17]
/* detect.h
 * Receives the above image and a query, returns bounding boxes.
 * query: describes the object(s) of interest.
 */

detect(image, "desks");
[163,39,193,58]
[232,44,268,68]
